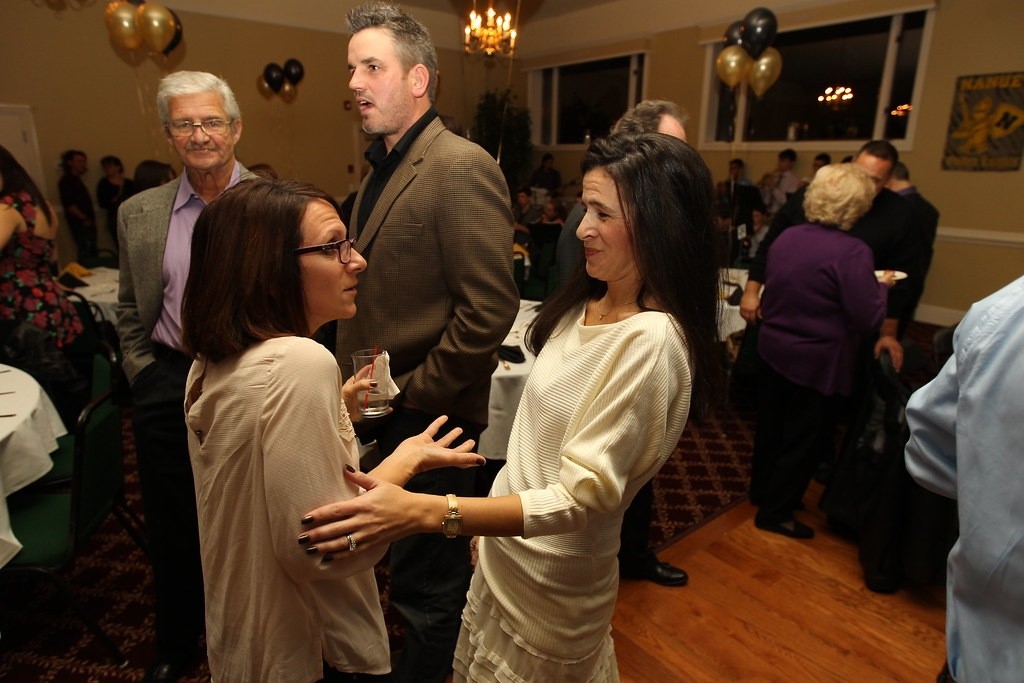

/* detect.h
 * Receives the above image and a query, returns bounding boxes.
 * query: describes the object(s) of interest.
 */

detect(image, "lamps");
[464,0,516,56]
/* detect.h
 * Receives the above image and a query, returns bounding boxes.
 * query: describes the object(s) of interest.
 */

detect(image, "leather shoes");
[620,560,687,585]
[143,644,202,683]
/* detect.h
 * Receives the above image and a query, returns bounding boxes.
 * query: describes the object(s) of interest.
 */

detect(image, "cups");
[351,348,390,419]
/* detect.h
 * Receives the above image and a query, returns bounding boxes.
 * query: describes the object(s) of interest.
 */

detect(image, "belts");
[153,343,193,369]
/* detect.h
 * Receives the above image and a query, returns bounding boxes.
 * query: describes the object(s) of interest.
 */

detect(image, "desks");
[64,266,119,323]
[0,362,68,571]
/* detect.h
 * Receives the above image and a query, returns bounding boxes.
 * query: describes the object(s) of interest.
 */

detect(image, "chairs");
[34,340,120,490]
[62,288,121,380]
[866,322,960,595]
[0,390,154,668]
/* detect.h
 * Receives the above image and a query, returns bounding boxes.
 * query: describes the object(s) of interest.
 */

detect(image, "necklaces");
[599,296,636,320]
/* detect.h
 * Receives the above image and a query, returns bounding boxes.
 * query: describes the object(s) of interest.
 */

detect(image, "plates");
[875,269,908,280]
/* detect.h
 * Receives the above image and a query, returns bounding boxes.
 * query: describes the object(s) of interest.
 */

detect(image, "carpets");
[0,320,941,683]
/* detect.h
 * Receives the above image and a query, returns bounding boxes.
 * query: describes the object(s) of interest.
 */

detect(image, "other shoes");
[749,488,805,512]
[686,404,705,429]
[755,512,814,537]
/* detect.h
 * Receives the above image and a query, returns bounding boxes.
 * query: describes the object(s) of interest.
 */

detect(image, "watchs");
[441,493,463,539]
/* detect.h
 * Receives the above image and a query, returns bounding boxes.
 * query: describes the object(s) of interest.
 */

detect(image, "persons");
[0,144,84,350]
[114,70,261,683]
[298,131,724,683]
[559,101,688,588]
[334,2,521,682]
[247,163,281,178]
[57,150,177,270]
[339,162,369,235]
[184,178,486,682]
[719,141,939,539]
[512,153,567,285]
[904,274,1023,683]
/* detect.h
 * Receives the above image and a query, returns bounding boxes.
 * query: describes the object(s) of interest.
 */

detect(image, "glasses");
[165,118,234,137]
[292,236,356,264]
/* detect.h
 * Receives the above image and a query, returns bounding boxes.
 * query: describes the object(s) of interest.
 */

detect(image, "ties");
[768,176,783,207]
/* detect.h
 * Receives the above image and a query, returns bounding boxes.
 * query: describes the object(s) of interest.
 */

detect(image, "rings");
[347,536,359,551]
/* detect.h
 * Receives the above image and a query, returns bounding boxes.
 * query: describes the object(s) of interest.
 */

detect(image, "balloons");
[716,6,782,99]
[256,58,304,104]
[104,0,184,63]
